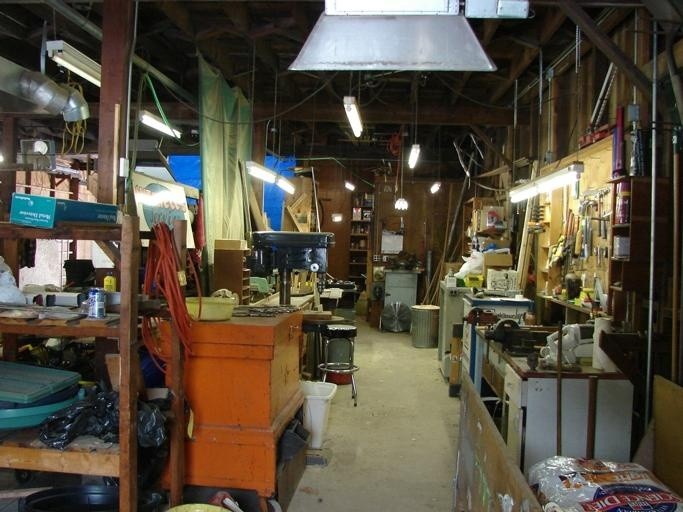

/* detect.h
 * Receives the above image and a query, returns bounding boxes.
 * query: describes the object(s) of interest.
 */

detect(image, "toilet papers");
[591,316,617,375]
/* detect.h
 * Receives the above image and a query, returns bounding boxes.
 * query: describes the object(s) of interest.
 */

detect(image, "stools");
[316,323,359,408]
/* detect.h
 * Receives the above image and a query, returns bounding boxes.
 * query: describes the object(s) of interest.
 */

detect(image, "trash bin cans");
[300,380,338,448]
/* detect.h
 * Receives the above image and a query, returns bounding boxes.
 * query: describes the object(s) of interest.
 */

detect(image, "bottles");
[614,182,630,223]
[80,288,105,318]
[277,266,292,306]
[471,232,478,250]
[552,271,608,321]
[448,269,454,277]
[103,272,117,292]
[355,290,371,316]
[496,311,537,327]
[350,223,367,249]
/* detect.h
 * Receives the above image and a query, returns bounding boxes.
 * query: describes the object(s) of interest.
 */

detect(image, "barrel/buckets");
[410,304,440,349]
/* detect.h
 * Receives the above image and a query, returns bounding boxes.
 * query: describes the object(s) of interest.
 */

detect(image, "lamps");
[342,179,442,210]
[341,70,368,141]
[136,64,184,139]
[506,24,586,205]
[402,85,423,173]
[243,34,276,186]
[43,1,101,85]
[276,83,297,196]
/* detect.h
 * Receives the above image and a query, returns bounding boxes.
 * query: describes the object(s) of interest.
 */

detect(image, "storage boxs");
[296,379,339,453]
[213,240,246,249]
[275,427,312,511]
[450,368,683,512]
[0,358,83,411]
[8,192,118,233]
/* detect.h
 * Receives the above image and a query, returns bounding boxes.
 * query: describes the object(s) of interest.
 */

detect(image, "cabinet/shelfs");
[0,215,192,511]
[345,192,373,293]
[161,303,308,512]
[439,132,670,476]
[212,248,251,305]
[382,271,417,308]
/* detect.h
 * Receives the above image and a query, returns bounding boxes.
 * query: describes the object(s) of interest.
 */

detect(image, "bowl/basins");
[185,296,233,320]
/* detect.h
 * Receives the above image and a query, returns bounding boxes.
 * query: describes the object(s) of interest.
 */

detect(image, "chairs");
[252,277,274,302]
[249,276,259,297]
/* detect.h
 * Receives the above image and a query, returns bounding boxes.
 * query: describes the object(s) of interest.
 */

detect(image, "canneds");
[86,287,105,321]
